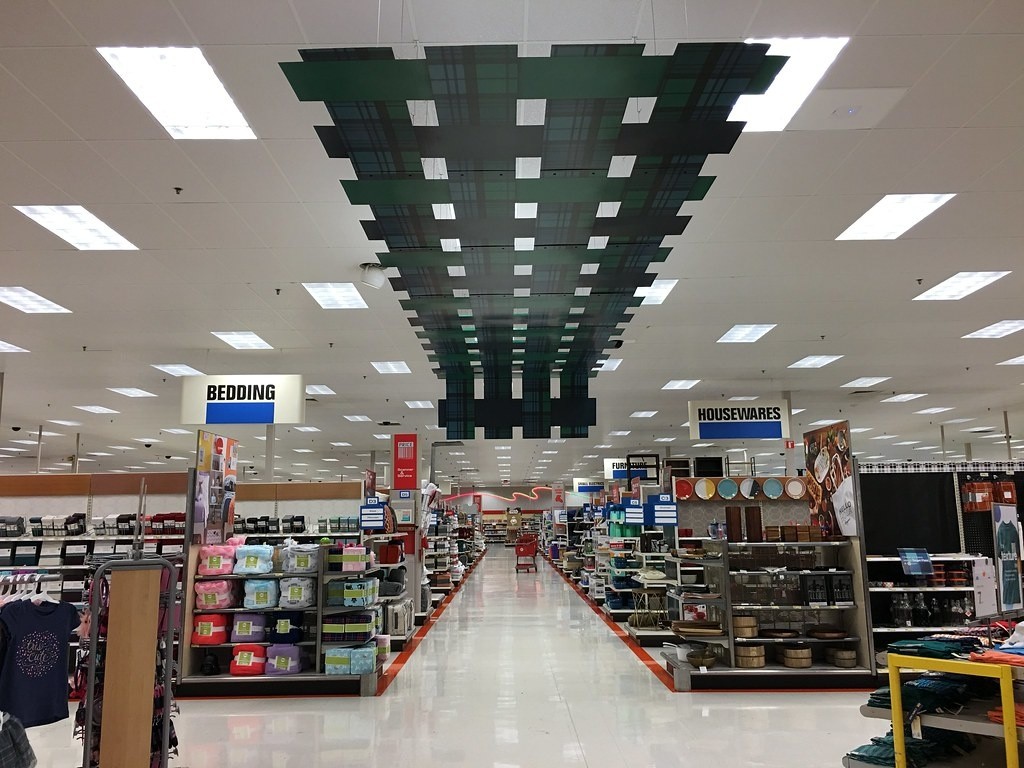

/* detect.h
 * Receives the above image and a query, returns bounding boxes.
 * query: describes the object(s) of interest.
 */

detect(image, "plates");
[784,478,807,498]
[814,449,830,483]
[717,478,738,499]
[836,431,846,452]
[763,478,783,498]
[695,478,715,500]
[675,479,692,500]
[739,478,760,499]
[825,477,832,491]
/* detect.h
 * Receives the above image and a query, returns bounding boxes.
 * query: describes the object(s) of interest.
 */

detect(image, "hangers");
[0,573,60,605]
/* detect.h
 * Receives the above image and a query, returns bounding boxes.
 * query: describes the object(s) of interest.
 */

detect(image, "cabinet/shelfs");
[374,484,486,653]
[537,420,1024,768]
[0,428,394,698]
[482,507,547,546]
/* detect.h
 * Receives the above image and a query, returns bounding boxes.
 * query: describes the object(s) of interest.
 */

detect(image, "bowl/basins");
[681,575,697,583]
[679,528,692,536]
[948,570,967,587]
[928,563,946,586]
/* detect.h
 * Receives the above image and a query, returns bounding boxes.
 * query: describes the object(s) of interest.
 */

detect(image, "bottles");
[889,593,971,626]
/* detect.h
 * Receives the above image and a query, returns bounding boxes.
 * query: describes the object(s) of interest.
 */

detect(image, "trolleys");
[515,530,538,572]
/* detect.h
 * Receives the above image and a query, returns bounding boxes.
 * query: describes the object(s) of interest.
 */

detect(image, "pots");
[661,641,706,662]
[686,649,718,668]
